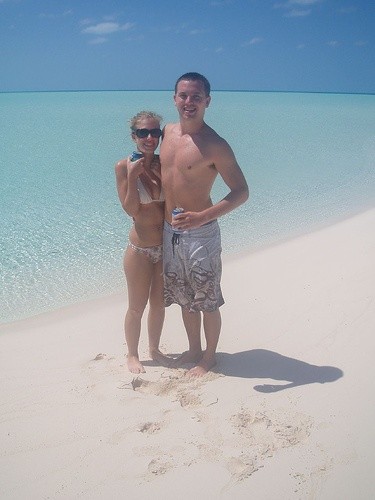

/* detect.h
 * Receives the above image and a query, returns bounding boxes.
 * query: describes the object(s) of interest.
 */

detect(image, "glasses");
[134,129,162,138]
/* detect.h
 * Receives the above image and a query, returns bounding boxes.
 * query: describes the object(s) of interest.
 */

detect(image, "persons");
[133,72,249,378]
[115,110,173,374]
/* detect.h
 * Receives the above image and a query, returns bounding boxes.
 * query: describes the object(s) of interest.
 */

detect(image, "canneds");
[130,151,144,162]
[171,207,185,234]
